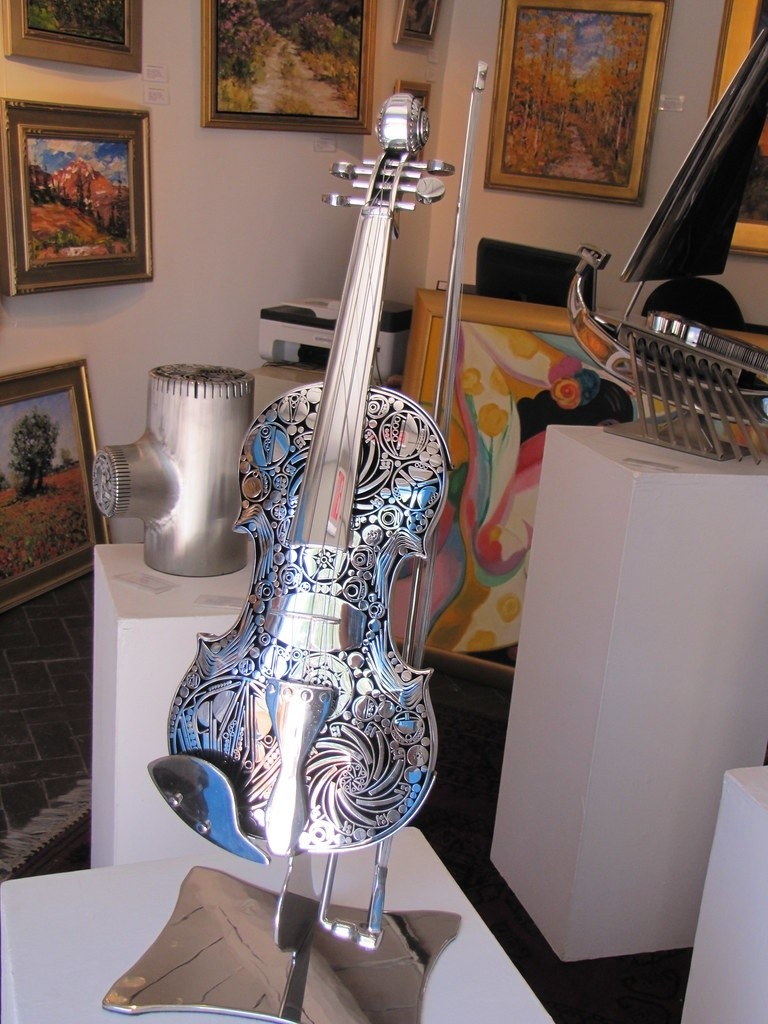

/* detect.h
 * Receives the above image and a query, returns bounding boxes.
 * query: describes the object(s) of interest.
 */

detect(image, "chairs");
[475,238,596,314]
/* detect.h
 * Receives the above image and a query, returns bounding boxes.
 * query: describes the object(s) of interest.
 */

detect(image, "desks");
[0,825,554,1024]
[88,540,258,864]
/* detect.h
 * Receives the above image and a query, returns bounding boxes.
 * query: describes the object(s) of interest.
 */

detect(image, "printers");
[257,297,414,380]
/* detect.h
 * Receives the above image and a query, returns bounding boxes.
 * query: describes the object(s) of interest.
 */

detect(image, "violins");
[149,91,457,863]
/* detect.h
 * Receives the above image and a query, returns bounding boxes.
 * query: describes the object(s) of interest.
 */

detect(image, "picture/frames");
[395,80,431,112]
[483,1,673,207]
[400,286,767,719]
[707,0,768,260]
[200,1,377,136]
[393,0,441,50]
[0,357,111,611]
[0,0,143,74]
[1,97,153,295]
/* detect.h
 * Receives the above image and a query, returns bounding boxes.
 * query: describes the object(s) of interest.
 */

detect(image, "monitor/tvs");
[476,238,597,311]
